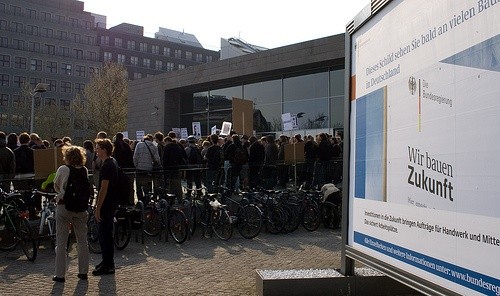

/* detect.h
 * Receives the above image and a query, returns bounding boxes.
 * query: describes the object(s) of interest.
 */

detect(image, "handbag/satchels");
[152,160,160,168]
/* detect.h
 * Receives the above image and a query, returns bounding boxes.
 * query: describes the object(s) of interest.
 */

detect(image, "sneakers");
[57,163,92,213]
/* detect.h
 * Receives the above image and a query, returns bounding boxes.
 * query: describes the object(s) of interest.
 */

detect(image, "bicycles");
[0,187,37,262]
[5,176,341,255]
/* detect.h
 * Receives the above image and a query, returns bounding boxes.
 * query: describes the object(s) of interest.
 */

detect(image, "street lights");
[28,81,49,137]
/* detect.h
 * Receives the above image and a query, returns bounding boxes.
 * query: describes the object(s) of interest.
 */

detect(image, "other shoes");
[53,275,66,283]
[78,273,88,280]
[95,259,104,269]
[92,266,115,276]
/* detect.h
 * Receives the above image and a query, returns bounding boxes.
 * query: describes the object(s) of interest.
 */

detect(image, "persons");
[0,131,343,231]
[194,125,200,135]
[92,139,121,276]
[52,146,89,281]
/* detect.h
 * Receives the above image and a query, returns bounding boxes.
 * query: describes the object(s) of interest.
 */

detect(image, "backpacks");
[189,146,204,165]
